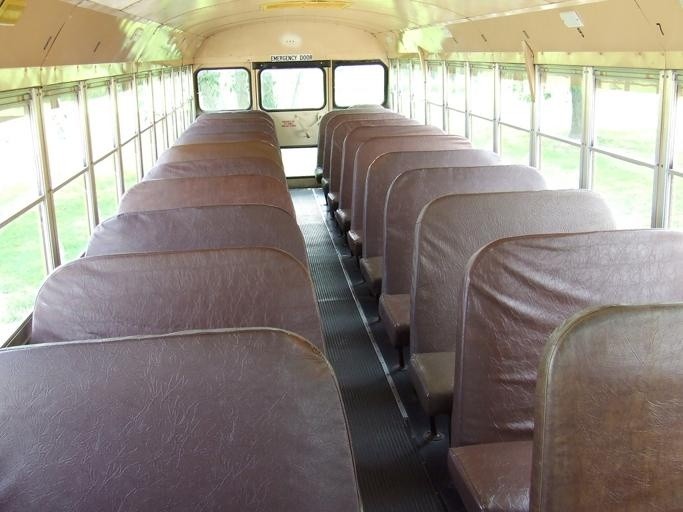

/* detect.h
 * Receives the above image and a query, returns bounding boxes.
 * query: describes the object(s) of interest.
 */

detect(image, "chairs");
[313,107,682,511]
[1,109,363,512]
